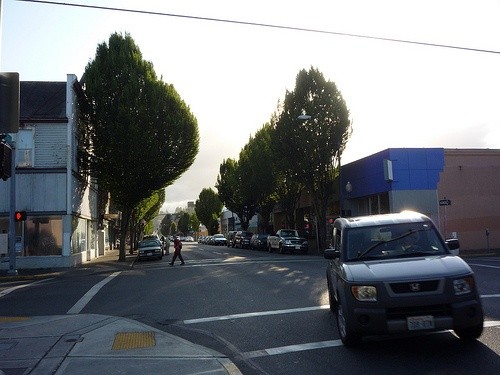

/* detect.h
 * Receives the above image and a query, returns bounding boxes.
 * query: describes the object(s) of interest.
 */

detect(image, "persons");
[116,237,120,249]
[169,234,185,265]
[160,236,170,256]
[398,229,424,252]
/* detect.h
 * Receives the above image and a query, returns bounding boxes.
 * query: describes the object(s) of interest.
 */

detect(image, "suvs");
[324,211,484,348]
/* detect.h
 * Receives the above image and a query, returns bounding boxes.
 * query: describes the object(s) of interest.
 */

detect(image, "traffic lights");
[15,211,27,221]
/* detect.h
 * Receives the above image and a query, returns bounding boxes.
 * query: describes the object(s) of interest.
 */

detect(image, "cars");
[138,236,163,262]
[198,231,271,250]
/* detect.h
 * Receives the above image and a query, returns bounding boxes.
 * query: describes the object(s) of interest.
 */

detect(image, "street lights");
[297,116,353,217]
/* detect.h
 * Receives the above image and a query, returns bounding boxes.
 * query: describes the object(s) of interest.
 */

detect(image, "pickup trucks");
[267,229,308,254]
